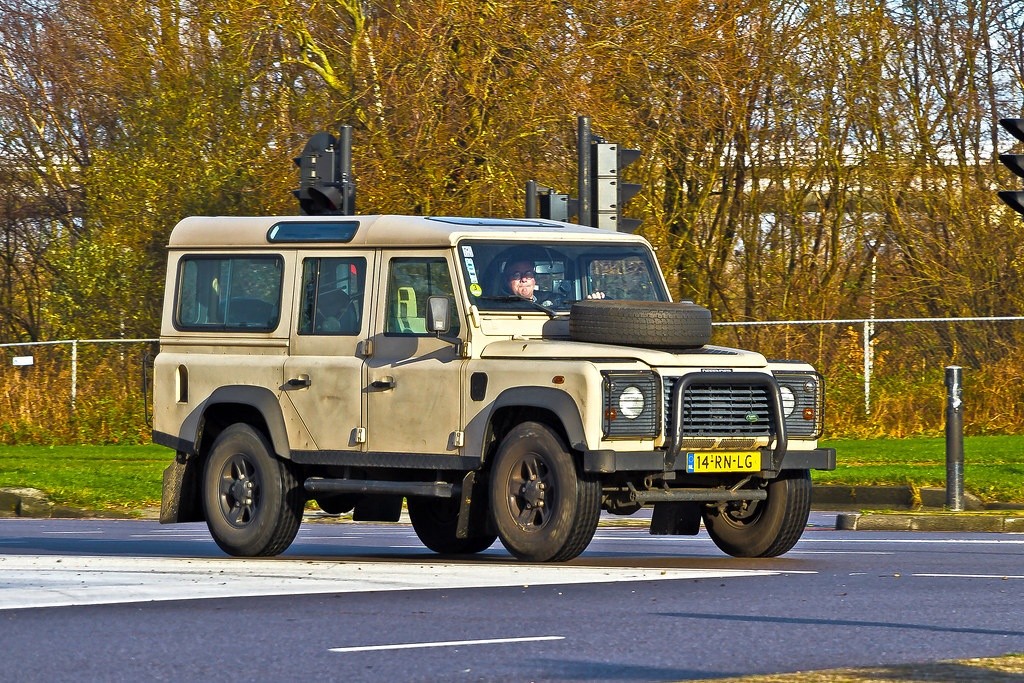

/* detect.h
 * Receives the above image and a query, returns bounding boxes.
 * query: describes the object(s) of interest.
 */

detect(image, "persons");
[500,258,614,307]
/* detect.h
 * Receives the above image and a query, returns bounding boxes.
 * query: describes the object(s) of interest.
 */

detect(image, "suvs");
[149,214,841,564]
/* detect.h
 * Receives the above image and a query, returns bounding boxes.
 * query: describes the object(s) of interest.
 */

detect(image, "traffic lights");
[596,142,645,234]
[999,113,1024,216]
[539,191,578,223]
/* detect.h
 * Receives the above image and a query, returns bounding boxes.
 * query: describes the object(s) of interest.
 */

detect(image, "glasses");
[508,270,534,280]
